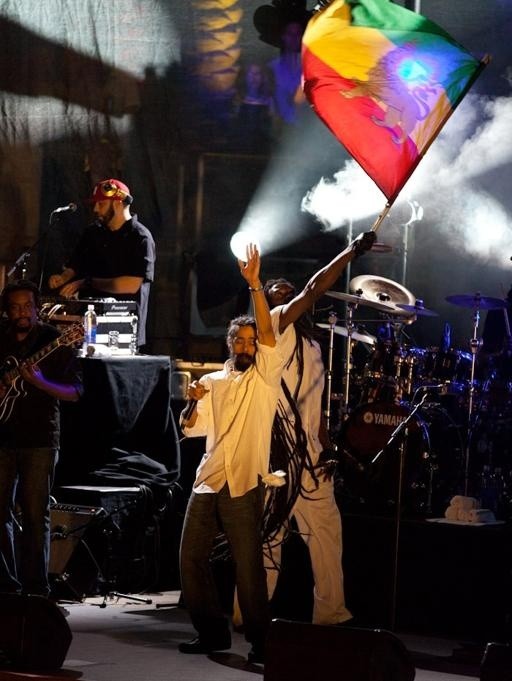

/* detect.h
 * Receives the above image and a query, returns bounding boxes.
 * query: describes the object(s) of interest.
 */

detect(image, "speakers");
[480,643,512,681]
[0,593,72,674]
[263,619,416,681]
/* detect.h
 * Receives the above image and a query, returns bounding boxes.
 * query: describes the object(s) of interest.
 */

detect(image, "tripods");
[60,499,152,608]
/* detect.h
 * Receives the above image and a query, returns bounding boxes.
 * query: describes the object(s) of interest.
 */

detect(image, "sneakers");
[179,633,231,652]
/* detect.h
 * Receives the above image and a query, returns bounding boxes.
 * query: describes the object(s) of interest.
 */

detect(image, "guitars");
[0,320,86,422]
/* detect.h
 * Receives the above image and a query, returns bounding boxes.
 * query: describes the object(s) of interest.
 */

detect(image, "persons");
[231,230,378,633]
[177,243,284,656]
[50,179,157,353]
[1,281,84,598]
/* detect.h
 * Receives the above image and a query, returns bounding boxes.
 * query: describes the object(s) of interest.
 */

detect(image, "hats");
[86,179,133,204]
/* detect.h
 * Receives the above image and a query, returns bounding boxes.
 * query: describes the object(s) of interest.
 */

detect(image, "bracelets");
[249,286,263,292]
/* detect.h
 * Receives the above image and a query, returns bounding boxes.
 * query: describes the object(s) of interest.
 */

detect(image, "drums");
[333,401,463,514]
[347,369,386,413]
[412,346,473,396]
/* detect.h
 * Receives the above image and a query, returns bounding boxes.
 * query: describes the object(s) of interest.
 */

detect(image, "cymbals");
[445,294,507,310]
[350,274,416,315]
[397,304,439,318]
[323,291,395,311]
[316,323,378,346]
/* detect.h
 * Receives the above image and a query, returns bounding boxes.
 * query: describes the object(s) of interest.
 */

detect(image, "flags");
[301,1,486,204]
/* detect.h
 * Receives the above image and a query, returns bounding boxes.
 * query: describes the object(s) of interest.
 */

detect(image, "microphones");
[359,464,373,476]
[179,400,199,428]
[51,202,77,214]
